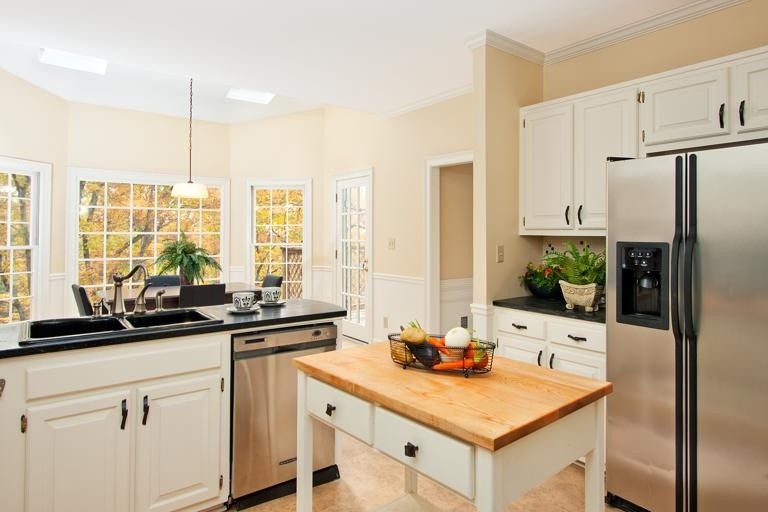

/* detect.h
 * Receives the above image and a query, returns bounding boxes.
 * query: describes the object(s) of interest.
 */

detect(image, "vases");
[517,260,562,293]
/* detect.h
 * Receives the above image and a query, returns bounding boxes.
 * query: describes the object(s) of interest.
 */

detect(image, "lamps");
[149,274,179,285]
[262,274,284,290]
[71,283,93,316]
[179,283,225,308]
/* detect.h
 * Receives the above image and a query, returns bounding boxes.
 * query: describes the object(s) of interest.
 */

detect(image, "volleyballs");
[405,340,440,368]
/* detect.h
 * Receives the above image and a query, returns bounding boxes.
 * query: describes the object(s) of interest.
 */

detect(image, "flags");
[524,279,561,298]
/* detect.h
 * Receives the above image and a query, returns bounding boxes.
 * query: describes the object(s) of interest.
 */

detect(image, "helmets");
[227,307,259,313]
[259,300,287,306]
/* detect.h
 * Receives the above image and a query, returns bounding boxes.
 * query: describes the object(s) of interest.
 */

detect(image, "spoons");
[465,342,489,369]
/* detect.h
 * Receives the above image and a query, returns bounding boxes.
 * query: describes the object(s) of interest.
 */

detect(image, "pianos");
[426,336,450,355]
[432,358,474,369]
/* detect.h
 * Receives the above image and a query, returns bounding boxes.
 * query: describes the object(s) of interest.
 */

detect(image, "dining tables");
[150,228,223,285]
[539,238,606,312]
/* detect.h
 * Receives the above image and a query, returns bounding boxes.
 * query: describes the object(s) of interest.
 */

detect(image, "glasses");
[171,78,209,200]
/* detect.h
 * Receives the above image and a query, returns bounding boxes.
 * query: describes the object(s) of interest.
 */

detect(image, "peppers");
[401,328,426,344]
[391,342,413,363]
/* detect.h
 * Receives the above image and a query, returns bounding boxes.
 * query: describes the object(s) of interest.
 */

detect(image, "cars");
[261,286,280,303]
[231,292,257,310]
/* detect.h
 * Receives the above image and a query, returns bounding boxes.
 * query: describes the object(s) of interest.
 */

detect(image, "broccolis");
[602,141,766,510]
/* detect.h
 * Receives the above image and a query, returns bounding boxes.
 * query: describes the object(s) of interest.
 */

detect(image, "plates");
[518,80,639,237]
[26,339,223,511]
[638,46,768,154]
[492,294,607,472]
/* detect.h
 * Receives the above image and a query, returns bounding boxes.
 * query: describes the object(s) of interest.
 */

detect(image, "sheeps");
[112,264,151,317]
